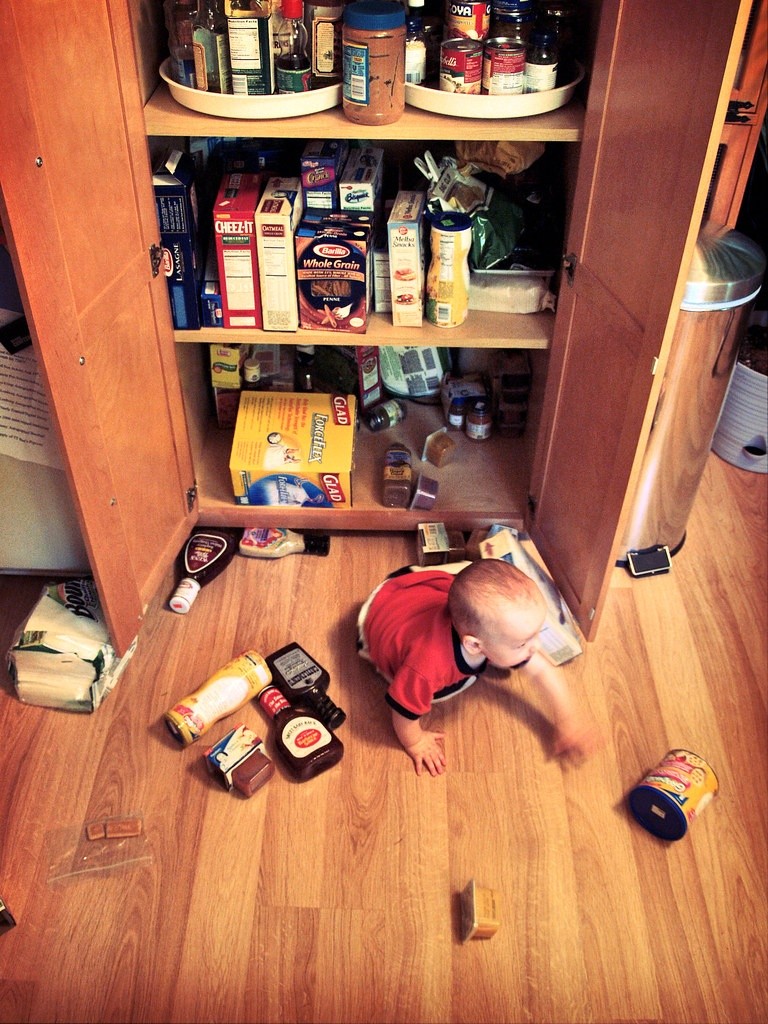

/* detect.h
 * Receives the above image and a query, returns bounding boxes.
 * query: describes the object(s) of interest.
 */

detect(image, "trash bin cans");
[612,220,767,578]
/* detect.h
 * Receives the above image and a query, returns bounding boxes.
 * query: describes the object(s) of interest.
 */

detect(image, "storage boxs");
[151,135,427,335]
[203,724,266,793]
[414,521,584,665]
[209,341,380,508]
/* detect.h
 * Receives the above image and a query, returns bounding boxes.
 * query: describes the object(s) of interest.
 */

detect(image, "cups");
[629,749,720,841]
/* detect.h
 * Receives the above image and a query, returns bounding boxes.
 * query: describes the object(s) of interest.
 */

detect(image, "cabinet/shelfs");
[0,0,768,654]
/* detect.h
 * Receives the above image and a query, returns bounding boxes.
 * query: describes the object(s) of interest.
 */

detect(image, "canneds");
[449,395,493,442]
[631,748,719,840]
[342,0,526,127]
[365,399,407,432]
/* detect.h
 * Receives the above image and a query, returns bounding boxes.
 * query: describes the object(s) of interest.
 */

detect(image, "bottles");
[383,443,410,508]
[240,345,317,392]
[448,397,468,430]
[169,528,239,614]
[425,210,473,328]
[163,641,346,781]
[164,0,570,126]
[466,398,492,441]
[239,526,331,560]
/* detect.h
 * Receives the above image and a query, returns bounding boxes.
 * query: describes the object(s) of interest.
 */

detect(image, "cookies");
[86,818,142,840]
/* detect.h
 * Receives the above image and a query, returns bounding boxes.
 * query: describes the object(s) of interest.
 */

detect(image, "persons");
[356,560,591,777]
